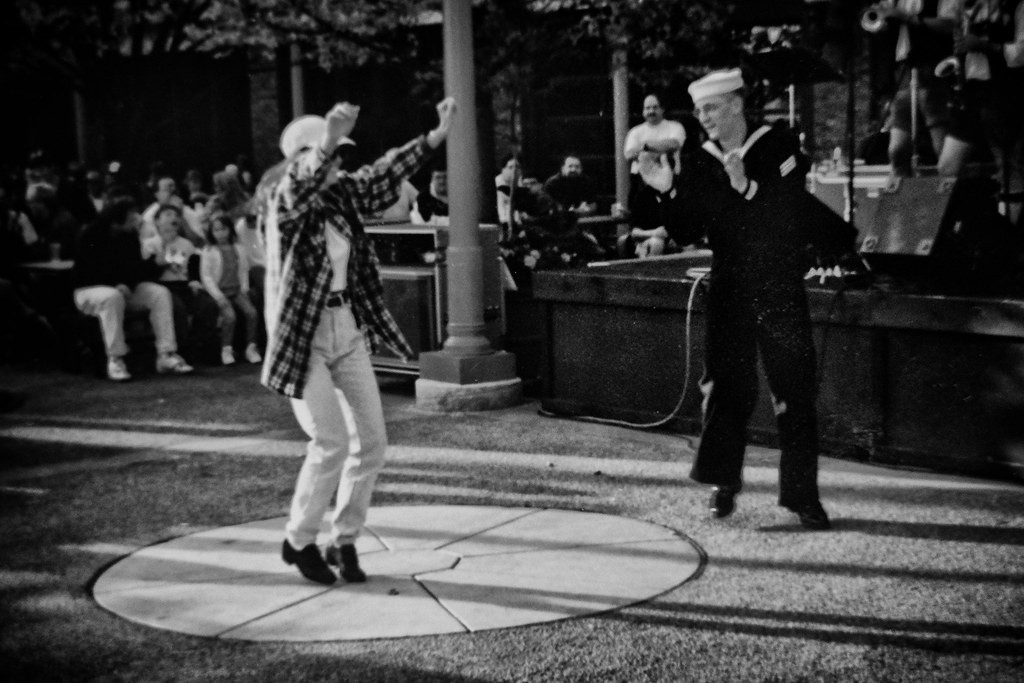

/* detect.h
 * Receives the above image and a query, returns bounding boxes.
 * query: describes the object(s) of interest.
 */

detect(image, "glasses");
[692,103,719,119]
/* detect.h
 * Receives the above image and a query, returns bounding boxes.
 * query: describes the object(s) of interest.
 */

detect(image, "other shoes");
[221,345,236,366]
[245,342,262,364]
[282,539,337,585]
[795,498,828,530]
[325,542,367,582]
[711,479,743,518]
[106,358,133,381]
[155,354,194,376]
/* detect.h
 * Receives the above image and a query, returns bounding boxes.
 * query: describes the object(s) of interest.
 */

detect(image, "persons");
[0,84,942,382]
[634,68,834,529]
[238,96,460,586]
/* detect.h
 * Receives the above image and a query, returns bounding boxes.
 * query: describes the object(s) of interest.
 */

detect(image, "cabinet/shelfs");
[363,223,504,376]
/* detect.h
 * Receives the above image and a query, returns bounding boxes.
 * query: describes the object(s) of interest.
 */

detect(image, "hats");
[279,115,357,162]
[687,68,744,104]
